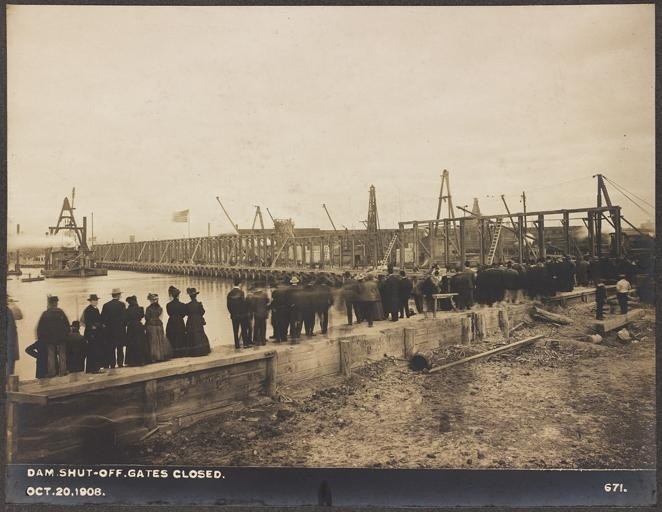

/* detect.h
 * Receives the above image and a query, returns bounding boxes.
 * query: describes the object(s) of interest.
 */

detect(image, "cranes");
[364,187,383,270]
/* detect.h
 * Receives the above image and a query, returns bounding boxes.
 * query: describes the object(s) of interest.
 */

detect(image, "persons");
[5,253,631,379]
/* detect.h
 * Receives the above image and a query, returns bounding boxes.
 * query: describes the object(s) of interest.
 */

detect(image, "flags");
[172,210,189,223]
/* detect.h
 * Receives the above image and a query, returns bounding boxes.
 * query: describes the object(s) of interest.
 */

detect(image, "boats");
[7,271,44,282]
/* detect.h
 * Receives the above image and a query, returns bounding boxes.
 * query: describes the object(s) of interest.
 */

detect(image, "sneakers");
[269,331,328,344]
[234,340,269,348]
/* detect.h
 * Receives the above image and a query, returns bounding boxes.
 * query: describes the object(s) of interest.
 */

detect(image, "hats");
[126,296,137,305]
[87,294,100,300]
[110,288,125,294]
[169,286,181,294]
[70,321,81,327]
[187,287,199,296]
[290,277,299,283]
[147,294,159,300]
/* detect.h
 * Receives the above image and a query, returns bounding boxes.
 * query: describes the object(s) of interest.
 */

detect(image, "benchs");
[433,293,458,312]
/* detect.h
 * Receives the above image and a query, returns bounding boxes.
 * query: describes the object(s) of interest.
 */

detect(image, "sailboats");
[41,188,107,276]
